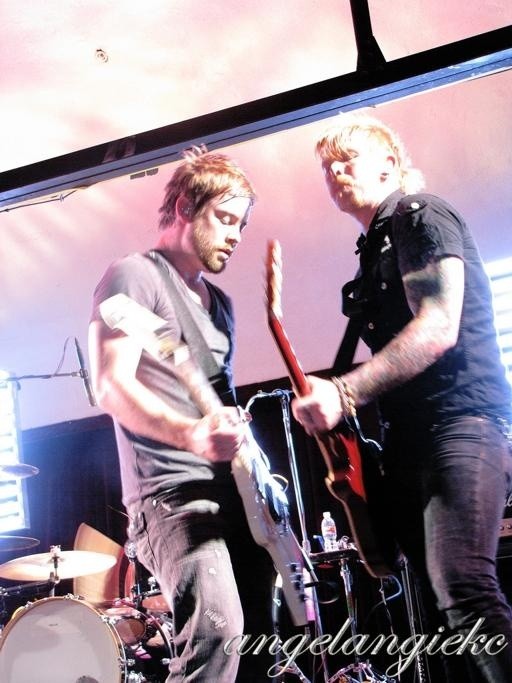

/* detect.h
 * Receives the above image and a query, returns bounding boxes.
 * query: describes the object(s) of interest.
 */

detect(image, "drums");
[125,538,175,615]
[0,593,174,683]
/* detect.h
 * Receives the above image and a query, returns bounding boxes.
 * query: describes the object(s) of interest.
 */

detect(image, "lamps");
[1,366,35,532]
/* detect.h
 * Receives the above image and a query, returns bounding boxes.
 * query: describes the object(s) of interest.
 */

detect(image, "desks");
[304,548,397,681]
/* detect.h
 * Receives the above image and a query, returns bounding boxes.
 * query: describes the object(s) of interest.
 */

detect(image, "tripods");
[326,561,395,683]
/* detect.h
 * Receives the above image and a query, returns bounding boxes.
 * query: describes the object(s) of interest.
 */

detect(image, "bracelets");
[327,374,357,419]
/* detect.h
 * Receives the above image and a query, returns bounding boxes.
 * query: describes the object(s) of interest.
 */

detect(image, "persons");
[289,108,510,681]
[85,140,279,683]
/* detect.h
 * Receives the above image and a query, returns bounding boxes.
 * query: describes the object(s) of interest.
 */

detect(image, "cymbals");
[1,462,40,482]
[0,550,117,582]
[0,535,39,551]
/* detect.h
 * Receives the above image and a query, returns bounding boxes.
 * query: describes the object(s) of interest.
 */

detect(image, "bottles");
[321,511,337,552]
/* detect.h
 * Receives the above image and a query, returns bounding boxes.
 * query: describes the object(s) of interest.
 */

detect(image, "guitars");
[98,292,321,626]
[266,238,401,578]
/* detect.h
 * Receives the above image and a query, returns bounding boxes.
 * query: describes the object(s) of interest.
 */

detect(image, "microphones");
[75,337,97,408]
